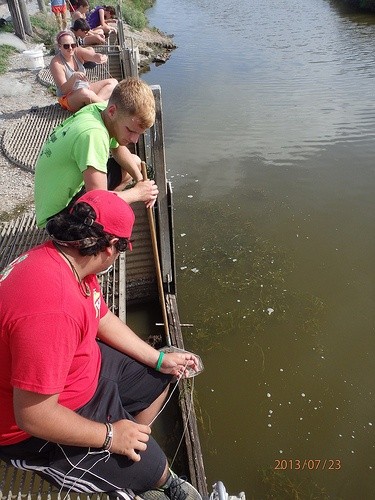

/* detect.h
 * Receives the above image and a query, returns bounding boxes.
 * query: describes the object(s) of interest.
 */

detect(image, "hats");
[69,190,135,252]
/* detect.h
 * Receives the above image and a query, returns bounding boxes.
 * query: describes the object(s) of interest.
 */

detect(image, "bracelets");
[101,421,113,450]
[155,351,164,372]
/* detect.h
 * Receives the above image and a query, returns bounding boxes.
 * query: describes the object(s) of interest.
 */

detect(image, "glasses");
[61,43,77,50]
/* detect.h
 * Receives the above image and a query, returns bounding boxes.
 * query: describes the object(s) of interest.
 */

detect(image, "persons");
[1,190,205,500]
[51,31,119,113]
[31,76,159,233]
[67,18,97,64]
[51,0,118,39]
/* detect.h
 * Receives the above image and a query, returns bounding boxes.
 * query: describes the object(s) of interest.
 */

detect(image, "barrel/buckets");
[23,49,45,71]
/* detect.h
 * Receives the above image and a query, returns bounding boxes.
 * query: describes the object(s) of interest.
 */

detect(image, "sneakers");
[159,467,203,500]
[142,490,171,500]
